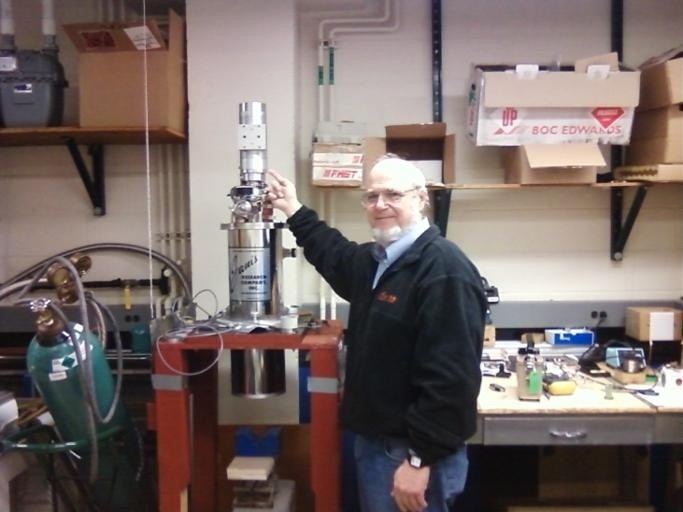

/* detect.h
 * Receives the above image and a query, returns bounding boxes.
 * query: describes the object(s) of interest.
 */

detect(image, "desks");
[614,366,683,511]
[463,362,655,511]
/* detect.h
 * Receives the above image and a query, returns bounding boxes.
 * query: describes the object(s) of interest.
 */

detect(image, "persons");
[266,157,486,511]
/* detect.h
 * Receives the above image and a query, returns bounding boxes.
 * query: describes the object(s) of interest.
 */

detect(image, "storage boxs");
[366,122,456,185]
[626,306,682,342]
[503,145,608,184]
[633,44,682,113]
[466,61,641,145]
[625,105,683,164]
[311,141,363,188]
[62,9,185,132]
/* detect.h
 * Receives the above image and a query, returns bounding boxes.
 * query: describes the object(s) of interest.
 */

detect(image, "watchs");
[405,446,431,468]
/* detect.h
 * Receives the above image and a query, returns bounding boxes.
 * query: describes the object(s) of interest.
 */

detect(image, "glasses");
[361,185,422,208]
